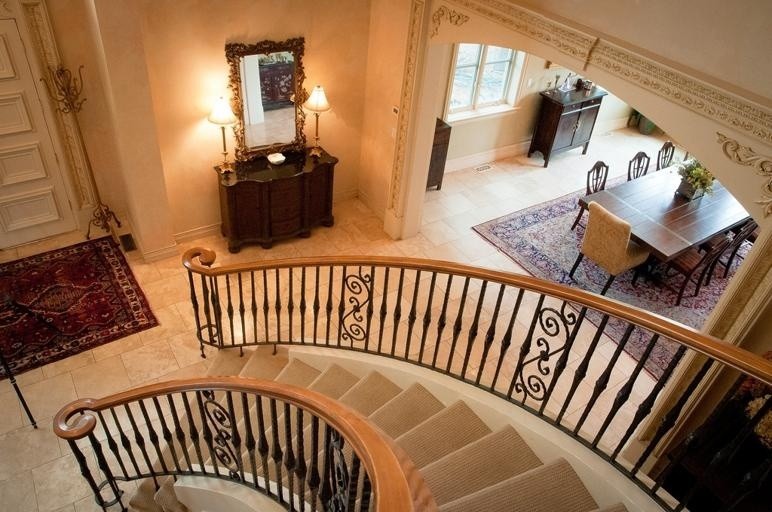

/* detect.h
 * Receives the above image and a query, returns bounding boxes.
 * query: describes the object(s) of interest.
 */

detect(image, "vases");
[676,179,699,200]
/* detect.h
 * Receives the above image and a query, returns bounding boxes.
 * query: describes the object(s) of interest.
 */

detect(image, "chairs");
[570,155,609,234]
[657,139,676,173]
[565,199,655,307]
[700,222,761,284]
[625,151,651,180]
[659,230,731,305]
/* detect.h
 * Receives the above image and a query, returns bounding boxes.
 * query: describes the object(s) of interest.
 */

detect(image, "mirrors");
[224,37,309,164]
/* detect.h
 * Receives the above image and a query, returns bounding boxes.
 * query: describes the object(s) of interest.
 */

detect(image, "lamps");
[206,96,239,175]
[302,81,334,160]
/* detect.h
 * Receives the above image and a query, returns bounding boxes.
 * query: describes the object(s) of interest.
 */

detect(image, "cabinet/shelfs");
[525,86,609,169]
[205,145,348,255]
[426,115,453,193]
[258,60,298,113]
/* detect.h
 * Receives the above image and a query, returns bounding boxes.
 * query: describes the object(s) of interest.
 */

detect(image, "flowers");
[671,151,714,194]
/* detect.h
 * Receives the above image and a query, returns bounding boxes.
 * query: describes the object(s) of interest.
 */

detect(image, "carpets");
[471,152,757,391]
[0,233,165,382]
